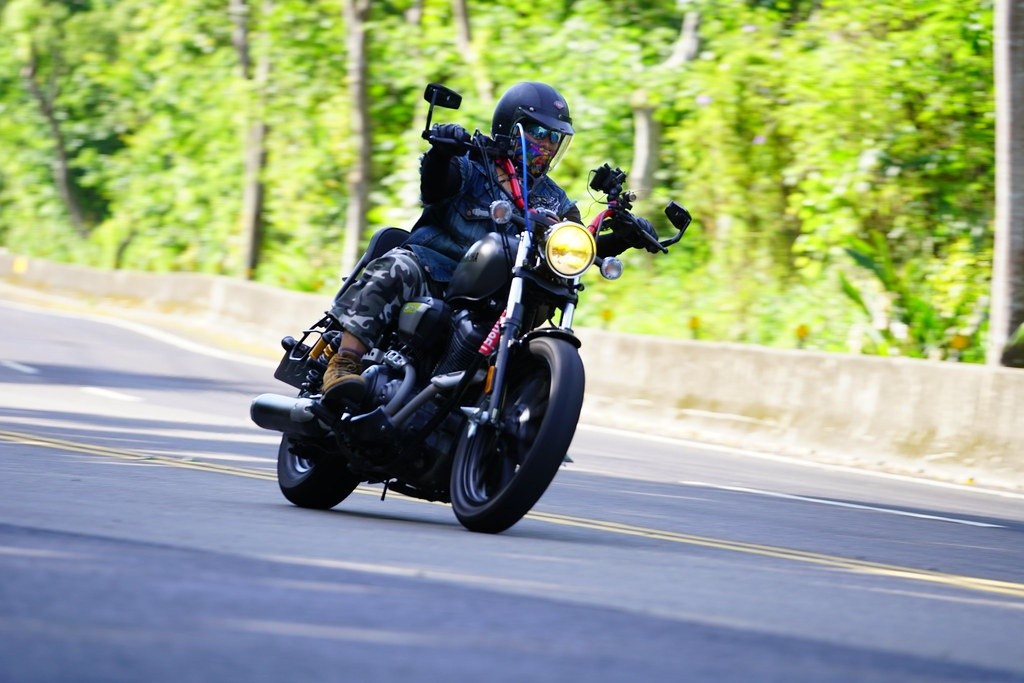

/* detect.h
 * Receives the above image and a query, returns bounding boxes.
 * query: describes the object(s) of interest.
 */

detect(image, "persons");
[318,82,661,409]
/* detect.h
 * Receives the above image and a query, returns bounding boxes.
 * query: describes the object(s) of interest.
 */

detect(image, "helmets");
[490,82,574,190]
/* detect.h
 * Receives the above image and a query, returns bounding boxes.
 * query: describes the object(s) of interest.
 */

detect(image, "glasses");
[524,122,562,145]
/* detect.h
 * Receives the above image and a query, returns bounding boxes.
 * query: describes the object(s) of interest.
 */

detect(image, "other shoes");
[320,352,367,414]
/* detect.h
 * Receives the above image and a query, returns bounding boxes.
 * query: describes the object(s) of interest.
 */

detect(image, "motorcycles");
[249,81,692,537]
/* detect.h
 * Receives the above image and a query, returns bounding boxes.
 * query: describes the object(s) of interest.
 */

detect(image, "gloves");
[433,123,468,157]
[620,215,660,254]
